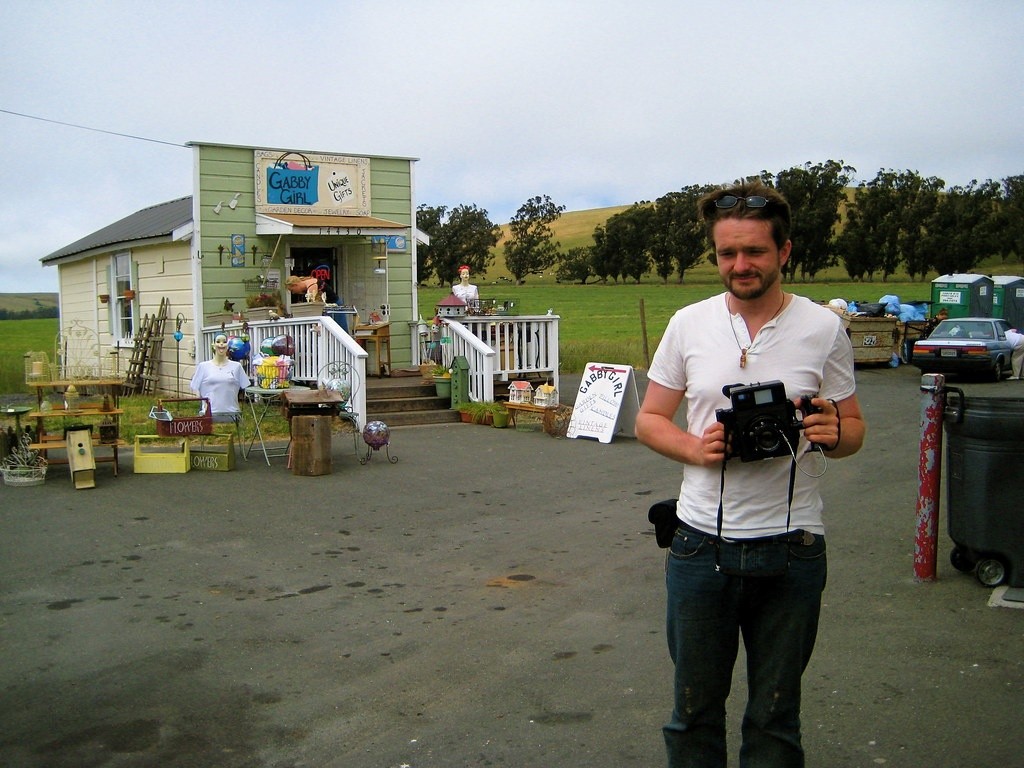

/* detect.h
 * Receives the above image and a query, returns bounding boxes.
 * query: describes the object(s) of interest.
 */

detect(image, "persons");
[284,277,350,333]
[191,334,251,419]
[451,266,480,315]
[919,309,948,340]
[1003,328,1024,380]
[635,181,867,768]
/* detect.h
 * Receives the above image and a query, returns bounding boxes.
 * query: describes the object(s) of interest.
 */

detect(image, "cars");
[913,317,1015,383]
[496,277,512,284]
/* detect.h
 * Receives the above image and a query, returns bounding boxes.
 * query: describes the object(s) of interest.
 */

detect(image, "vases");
[418,365,439,382]
[99,295,109,303]
[122,290,136,300]
[434,382,451,398]
[290,302,325,318]
[247,308,278,321]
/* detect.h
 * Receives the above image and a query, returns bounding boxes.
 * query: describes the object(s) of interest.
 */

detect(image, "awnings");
[417,228,429,247]
[253,213,412,294]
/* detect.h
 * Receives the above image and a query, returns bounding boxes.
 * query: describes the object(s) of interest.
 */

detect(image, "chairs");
[969,331,983,337]
[952,326,966,338]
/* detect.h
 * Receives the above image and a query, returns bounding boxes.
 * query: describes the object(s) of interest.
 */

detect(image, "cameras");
[714,380,823,465]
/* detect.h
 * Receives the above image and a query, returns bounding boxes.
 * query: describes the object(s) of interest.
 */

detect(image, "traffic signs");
[566,362,632,444]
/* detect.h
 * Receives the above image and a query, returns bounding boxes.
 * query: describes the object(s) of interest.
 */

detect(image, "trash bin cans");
[945,397,1024,586]
[807,298,932,368]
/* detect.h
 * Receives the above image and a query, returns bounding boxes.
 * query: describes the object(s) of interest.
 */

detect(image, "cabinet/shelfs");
[25,379,125,477]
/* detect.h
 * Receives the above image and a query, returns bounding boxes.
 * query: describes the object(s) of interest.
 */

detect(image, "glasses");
[714,194,775,208]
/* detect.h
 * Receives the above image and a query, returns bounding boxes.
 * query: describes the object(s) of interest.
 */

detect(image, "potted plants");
[454,400,514,428]
[430,365,451,383]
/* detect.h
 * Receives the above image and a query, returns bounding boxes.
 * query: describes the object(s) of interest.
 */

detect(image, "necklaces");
[728,290,784,369]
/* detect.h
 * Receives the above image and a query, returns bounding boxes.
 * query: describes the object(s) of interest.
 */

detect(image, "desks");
[354,321,392,378]
[244,386,310,466]
[503,401,546,430]
